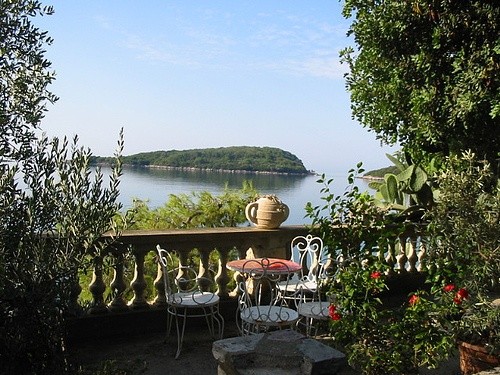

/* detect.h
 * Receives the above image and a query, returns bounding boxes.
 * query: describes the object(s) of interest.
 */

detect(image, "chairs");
[157,234,362,360]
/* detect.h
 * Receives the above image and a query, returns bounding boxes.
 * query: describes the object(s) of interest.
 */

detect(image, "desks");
[225,258,303,306]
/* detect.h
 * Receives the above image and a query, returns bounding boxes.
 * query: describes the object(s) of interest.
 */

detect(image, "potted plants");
[457,298,500,375]
